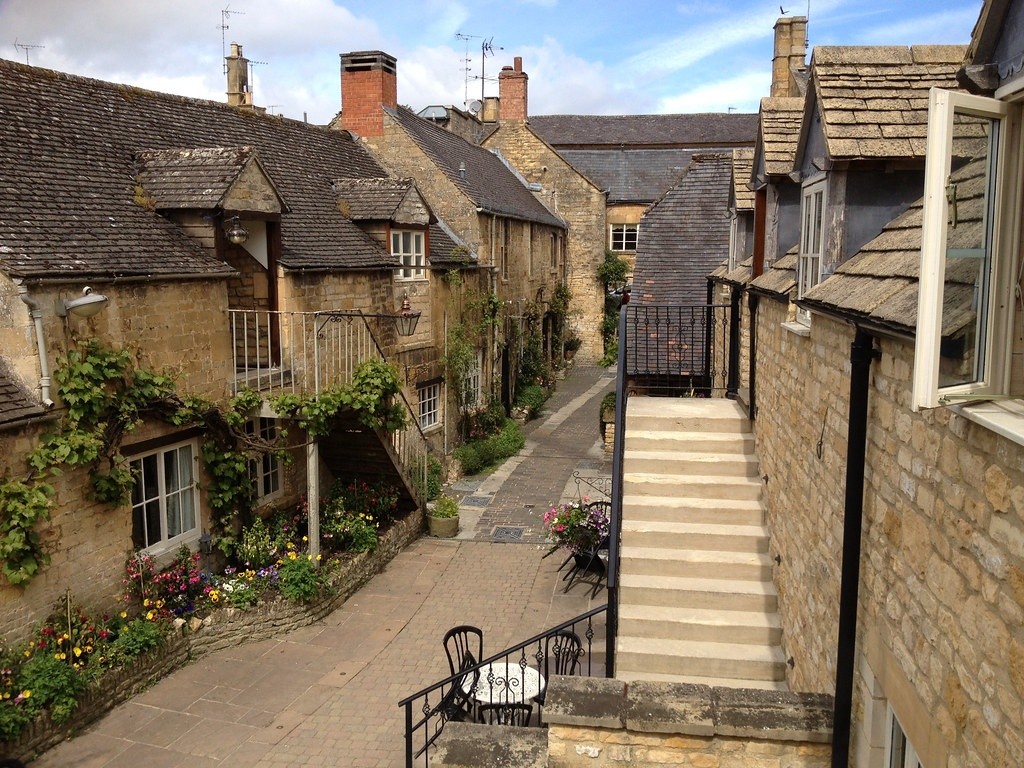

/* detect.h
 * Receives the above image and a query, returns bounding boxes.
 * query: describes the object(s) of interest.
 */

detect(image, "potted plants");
[425,491,461,539]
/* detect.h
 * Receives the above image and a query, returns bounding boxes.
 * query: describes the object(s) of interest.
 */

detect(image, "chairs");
[442,624,484,714]
[403,649,481,759]
[541,501,611,600]
[478,702,533,727]
[532,630,582,706]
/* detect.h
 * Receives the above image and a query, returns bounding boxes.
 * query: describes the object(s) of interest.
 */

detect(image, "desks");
[580,516,610,537]
[460,662,546,726]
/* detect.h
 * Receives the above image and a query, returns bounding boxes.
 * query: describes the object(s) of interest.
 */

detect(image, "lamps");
[53,286,109,318]
[314,292,422,340]
[224,215,250,244]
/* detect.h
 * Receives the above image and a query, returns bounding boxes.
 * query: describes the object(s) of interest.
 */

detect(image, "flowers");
[541,496,609,556]
